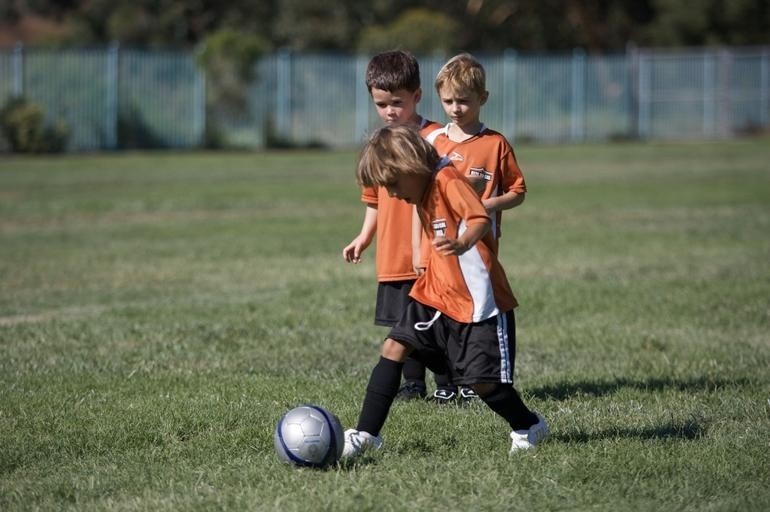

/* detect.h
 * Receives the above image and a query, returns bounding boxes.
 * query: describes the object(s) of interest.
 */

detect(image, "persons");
[410,53,526,404]
[342,49,444,402]
[333,125,551,460]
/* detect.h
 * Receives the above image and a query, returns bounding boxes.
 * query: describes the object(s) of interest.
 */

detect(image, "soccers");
[274,405,345,469]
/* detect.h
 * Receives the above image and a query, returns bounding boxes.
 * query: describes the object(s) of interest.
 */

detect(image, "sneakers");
[399,381,480,407]
[342,428,383,462]
[510,411,550,453]
